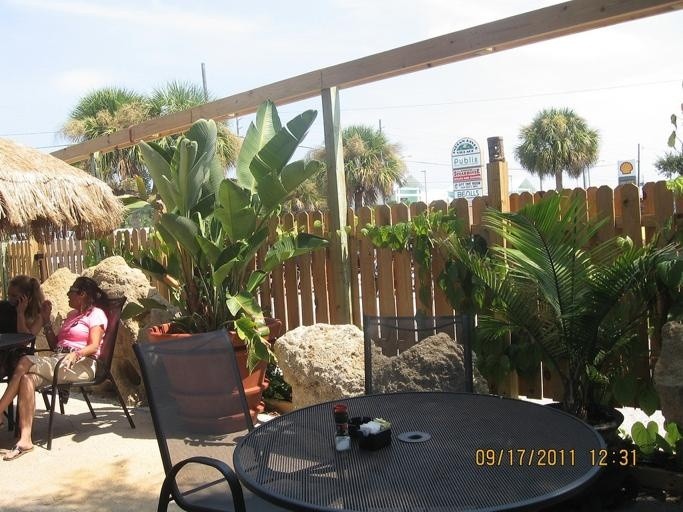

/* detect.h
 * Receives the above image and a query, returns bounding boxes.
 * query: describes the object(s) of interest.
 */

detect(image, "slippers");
[3,443,36,461]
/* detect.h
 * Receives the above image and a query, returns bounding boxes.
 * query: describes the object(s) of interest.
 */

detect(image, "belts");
[54,346,96,360]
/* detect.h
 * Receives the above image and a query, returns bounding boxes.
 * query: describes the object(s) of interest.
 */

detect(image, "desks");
[0,332,37,438]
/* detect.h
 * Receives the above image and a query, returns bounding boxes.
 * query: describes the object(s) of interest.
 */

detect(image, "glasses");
[68,286,79,293]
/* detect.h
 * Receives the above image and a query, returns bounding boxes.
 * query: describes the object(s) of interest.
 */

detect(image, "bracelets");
[74,350,81,361]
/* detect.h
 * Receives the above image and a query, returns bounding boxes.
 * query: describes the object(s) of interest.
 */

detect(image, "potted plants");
[86,99,327,436]
[434,192,683,494]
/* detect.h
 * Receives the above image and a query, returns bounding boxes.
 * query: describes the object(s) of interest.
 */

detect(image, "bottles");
[332,404,352,451]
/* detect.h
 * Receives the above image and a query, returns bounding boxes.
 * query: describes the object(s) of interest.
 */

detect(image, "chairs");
[132,330,351,512]
[363,312,473,395]
[0,340,64,425]
[14,296,135,450]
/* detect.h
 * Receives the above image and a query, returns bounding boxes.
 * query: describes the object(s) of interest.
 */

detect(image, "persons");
[0,274,45,380]
[0,276,111,462]
